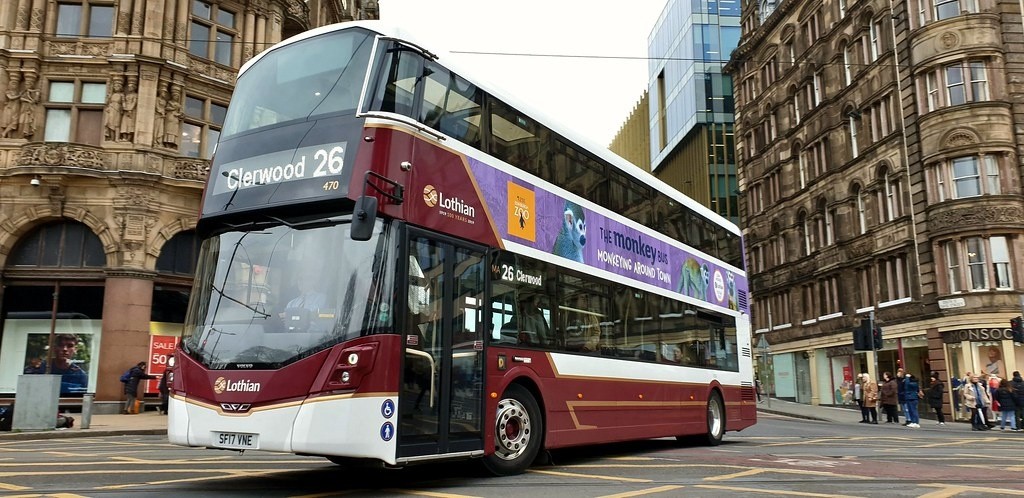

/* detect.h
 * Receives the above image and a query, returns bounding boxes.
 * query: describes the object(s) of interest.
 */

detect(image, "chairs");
[273,122,737,380]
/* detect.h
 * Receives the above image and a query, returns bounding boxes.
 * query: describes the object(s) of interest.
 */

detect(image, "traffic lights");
[1010,316,1024,346]
[872,326,884,350]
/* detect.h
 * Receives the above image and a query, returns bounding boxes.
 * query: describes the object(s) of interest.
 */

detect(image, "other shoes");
[124,410,129,414]
[903,421,910,426]
[859,419,869,423]
[886,422,891,424]
[1011,428,1017,430]
[157,407,162,414]
[1001,427,1004,430]
[987,426,994,430]
[972,426,986,431]
[892,422,899,424]
[869,421,878,424]
[936,422,946,428]
[131,410,137,414]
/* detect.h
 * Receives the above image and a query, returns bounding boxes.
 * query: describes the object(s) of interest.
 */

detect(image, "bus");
[166,20,760,480]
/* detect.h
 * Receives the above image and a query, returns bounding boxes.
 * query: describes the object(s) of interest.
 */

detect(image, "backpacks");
[120,369,139,381]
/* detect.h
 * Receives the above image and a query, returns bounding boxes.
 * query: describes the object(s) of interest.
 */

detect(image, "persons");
[982,347,1006,378]
[158,369,170,415]
[753,372,764,403]
[122,361,161,414]
[286,248,732,372]
[25,333,88,394]
[852,367,1024,429]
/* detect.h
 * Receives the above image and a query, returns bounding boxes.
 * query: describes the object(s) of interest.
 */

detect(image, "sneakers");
[907,422,920,428]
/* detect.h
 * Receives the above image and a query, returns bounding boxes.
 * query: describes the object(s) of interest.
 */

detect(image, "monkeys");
[552,199,587,264]
[677,258,710,301]
[725,270,738,312]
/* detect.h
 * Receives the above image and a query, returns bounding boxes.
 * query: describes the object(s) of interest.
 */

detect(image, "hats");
[930,372,939,378]
